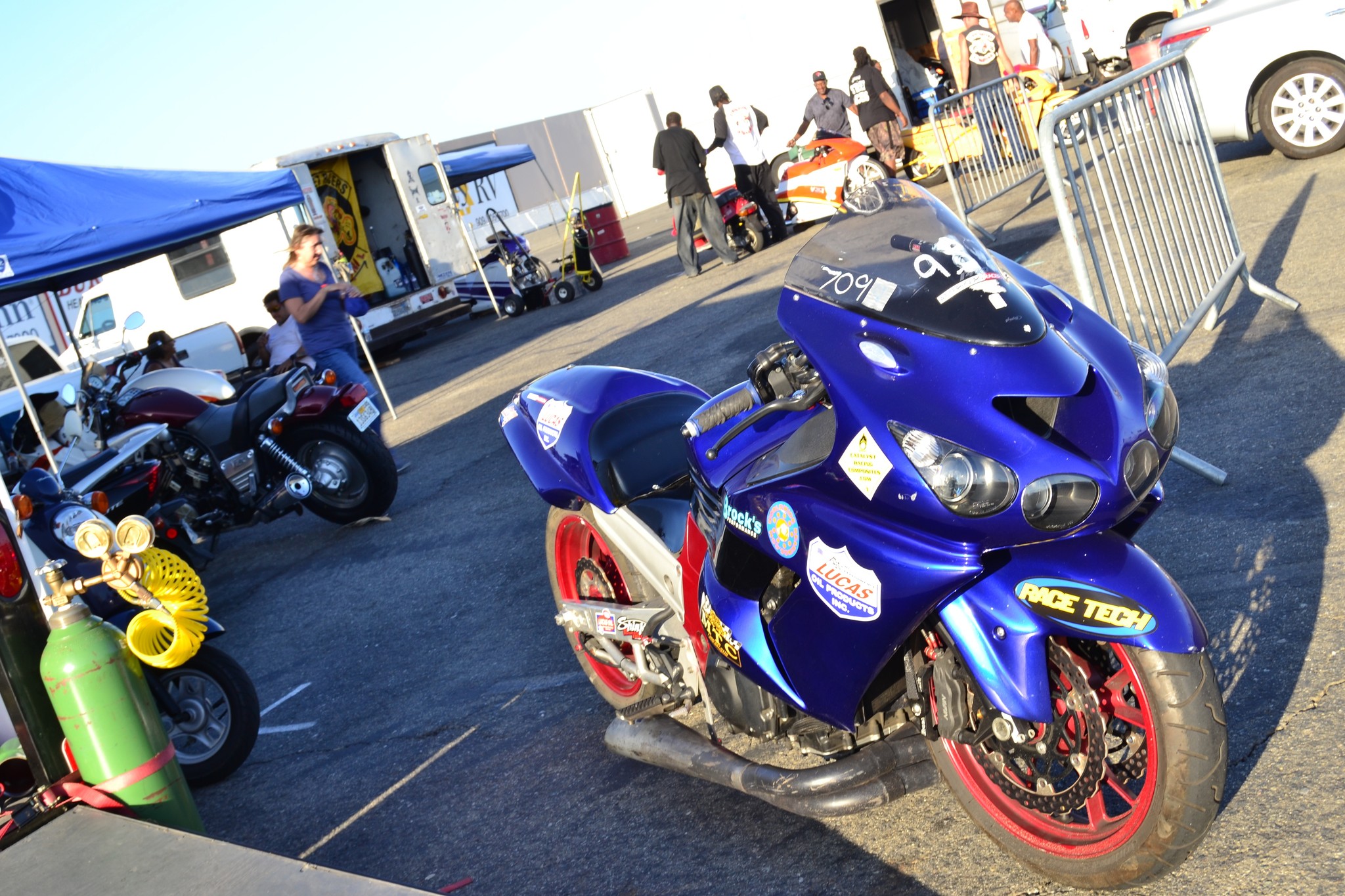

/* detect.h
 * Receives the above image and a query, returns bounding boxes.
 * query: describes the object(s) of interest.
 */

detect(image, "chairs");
[239,355,323,381]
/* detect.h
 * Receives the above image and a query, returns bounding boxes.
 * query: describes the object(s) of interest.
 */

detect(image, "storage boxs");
[911,83,949,119]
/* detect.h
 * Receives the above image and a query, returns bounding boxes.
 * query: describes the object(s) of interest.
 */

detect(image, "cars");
[1155,1,1345,160]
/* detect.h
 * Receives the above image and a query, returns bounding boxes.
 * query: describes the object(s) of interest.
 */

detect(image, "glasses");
[825,97,833,106]
[267,304,282,313]
[822,99,830,110]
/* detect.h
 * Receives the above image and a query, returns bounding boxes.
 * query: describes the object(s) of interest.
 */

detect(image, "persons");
[655,111,742,278]
[279,223,412,474]
[141,330,223,382]
[958,1,1034,176]
[848,47,908,179]
[238,289,308,401]
[703,85,789,245]
[787,71,859,148]
[871,59,882,72]
[1003,0,1061,94]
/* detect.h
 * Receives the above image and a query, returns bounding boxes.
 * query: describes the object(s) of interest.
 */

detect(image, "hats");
[709,85,726,103]
[951,2,988,20]
[813,70,826,82]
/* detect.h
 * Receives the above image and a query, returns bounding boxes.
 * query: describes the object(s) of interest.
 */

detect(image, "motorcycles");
[895,64,1093,181]
[63,311,398,526]
[494,176,1227,891]
[0,422,260,786]
[659,138,890,257]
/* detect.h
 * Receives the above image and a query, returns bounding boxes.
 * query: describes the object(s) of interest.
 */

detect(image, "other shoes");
[1017,155,1030,165]
[978,169,998,178]
[386,448,413,471]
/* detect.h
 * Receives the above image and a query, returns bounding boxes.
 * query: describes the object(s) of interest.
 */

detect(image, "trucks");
[58,132,473,393]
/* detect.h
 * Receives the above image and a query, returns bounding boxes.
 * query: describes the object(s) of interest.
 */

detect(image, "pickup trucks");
[0,321,249,417]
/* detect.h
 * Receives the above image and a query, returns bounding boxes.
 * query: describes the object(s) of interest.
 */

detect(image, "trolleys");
[485,207,555,317]
[553,173,604,304]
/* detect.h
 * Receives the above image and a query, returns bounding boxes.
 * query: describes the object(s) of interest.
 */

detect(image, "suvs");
[1024,0,1213,85]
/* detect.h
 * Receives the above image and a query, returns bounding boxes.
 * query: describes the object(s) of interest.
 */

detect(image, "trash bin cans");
[577,201,630,265]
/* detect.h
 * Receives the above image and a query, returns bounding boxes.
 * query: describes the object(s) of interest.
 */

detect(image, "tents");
[417,142,604,320]
[1,156,405,509]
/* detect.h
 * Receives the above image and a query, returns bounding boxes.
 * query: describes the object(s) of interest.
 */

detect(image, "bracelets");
[289,353,302,368]
[961,87,968,92]
[705,149,709,154]
[792,137,797,142]
